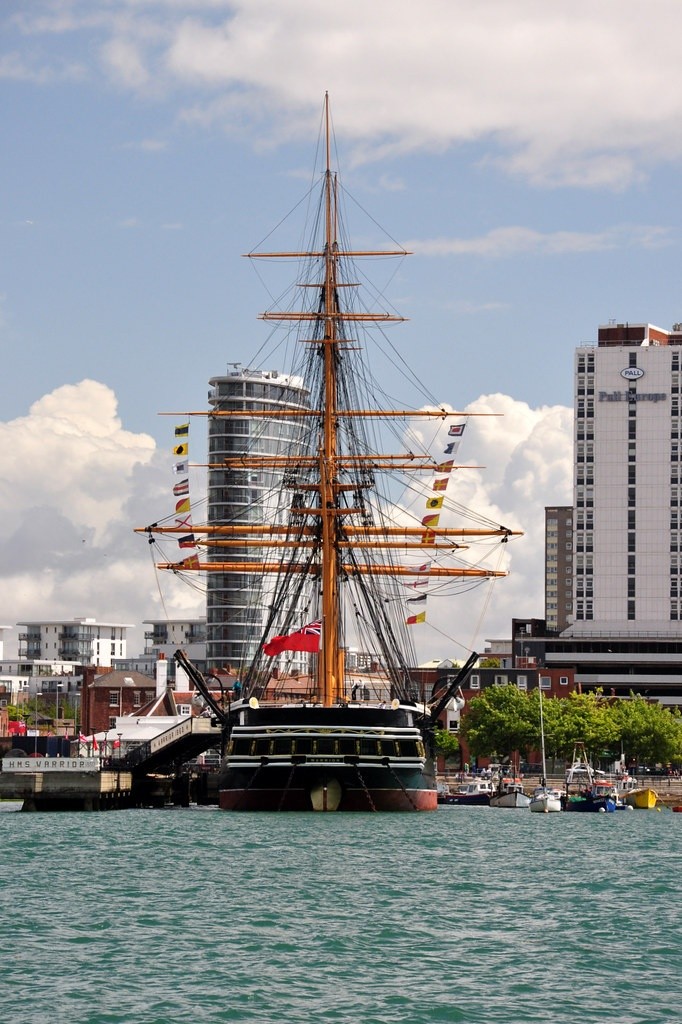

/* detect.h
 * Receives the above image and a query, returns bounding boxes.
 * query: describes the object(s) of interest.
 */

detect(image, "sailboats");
[529,673,562,814]
[136,91,527,812]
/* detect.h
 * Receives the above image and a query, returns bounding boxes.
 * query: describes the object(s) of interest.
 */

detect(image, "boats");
[564,796,617,813]
[437,764,524,805]
[489,792,532,808]
[618,787,658,809]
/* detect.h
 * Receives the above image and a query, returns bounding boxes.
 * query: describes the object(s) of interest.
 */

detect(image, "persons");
[542,778,546,787]
[668,768,680,779]
[455,763,524,783]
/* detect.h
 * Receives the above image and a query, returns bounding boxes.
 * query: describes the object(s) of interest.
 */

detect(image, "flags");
[262,619,323,656]
[47,731,121,751]
[405,423,467,627]
[170,424,201,570]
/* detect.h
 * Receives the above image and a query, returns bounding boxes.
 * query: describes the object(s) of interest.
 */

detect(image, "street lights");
[74,693,82,736]
[56,683,64,735]
[35,692,43,756]
